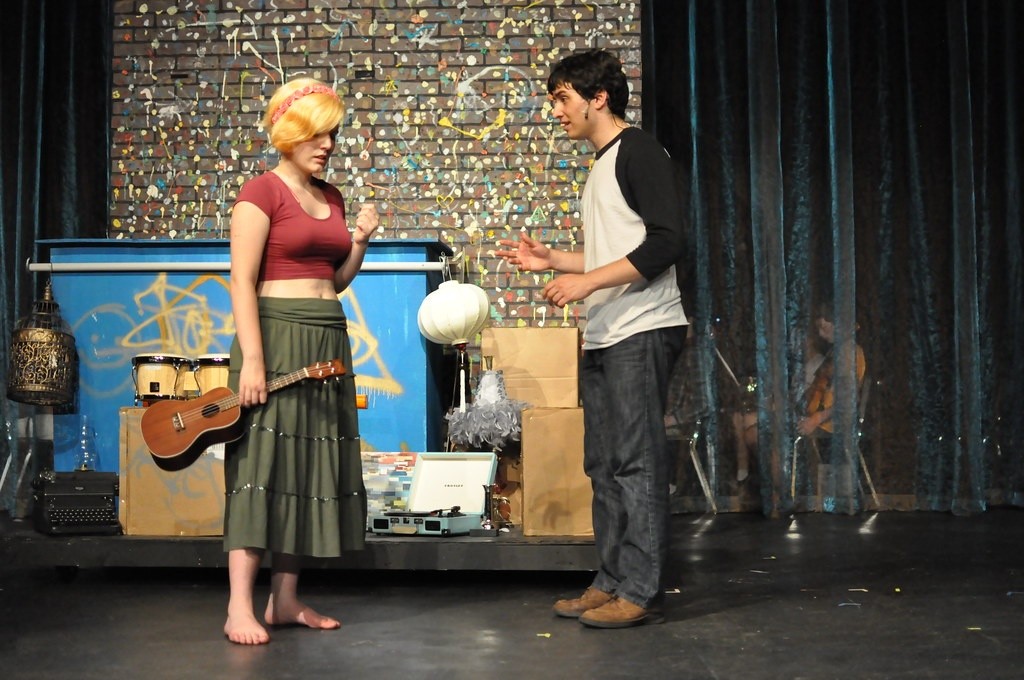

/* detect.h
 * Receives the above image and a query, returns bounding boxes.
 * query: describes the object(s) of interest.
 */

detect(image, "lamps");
[444,356,533,529]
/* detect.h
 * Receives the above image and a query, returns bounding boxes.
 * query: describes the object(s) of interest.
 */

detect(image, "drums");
[130,352,192,403]
[193,352,232,396]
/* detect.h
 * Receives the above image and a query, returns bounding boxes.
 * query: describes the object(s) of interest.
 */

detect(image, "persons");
[494,49,689,628]
[664,297,867,514]
[223,78,382,646]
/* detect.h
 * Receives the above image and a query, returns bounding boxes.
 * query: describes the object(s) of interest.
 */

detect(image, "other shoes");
[553,586,616,619]
[579,594,665,628]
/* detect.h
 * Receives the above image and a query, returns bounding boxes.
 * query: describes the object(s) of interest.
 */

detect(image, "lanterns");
[418,280,490,413]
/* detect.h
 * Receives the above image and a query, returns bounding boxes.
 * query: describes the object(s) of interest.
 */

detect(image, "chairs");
[665,418,718,515]
[790,377,881,519]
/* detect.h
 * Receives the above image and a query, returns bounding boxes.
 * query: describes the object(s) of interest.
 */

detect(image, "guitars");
[140,358,347,472]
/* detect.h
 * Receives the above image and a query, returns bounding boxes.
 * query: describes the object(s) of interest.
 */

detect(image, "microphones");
[584,99,590,119]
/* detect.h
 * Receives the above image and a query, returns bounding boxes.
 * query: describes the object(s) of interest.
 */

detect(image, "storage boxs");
[480,327,595,537]
[368,452,502,535]
[118,407,226,535]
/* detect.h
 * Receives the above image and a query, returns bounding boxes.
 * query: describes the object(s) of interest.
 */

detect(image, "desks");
[32,238,455,517]
